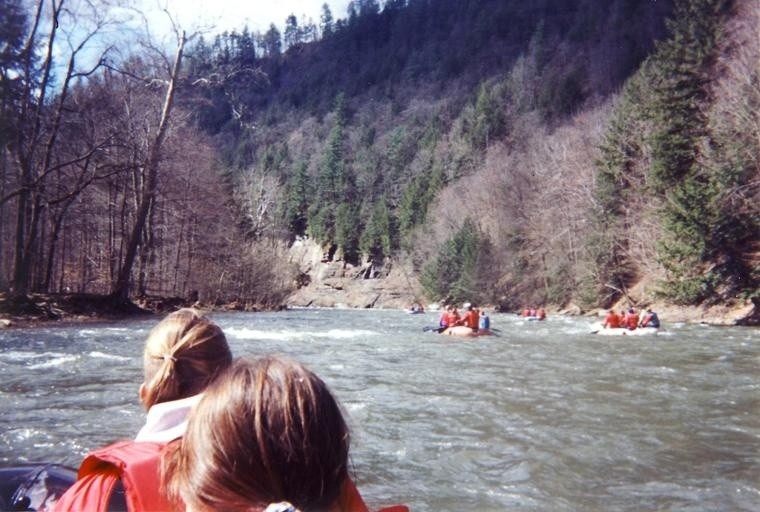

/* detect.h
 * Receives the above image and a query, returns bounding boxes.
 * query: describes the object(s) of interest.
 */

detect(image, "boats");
[439,325,493,338]
[592,322,664,337]
[406,307,424,315]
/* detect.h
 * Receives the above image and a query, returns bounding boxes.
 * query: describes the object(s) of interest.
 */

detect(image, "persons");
[440,301,490,332]
[50,306,368,512]
[169,353,351,512]
[523,306,529,317]
[537,308,546,321]
[601,307,661,331]
[529,306,536,316]
